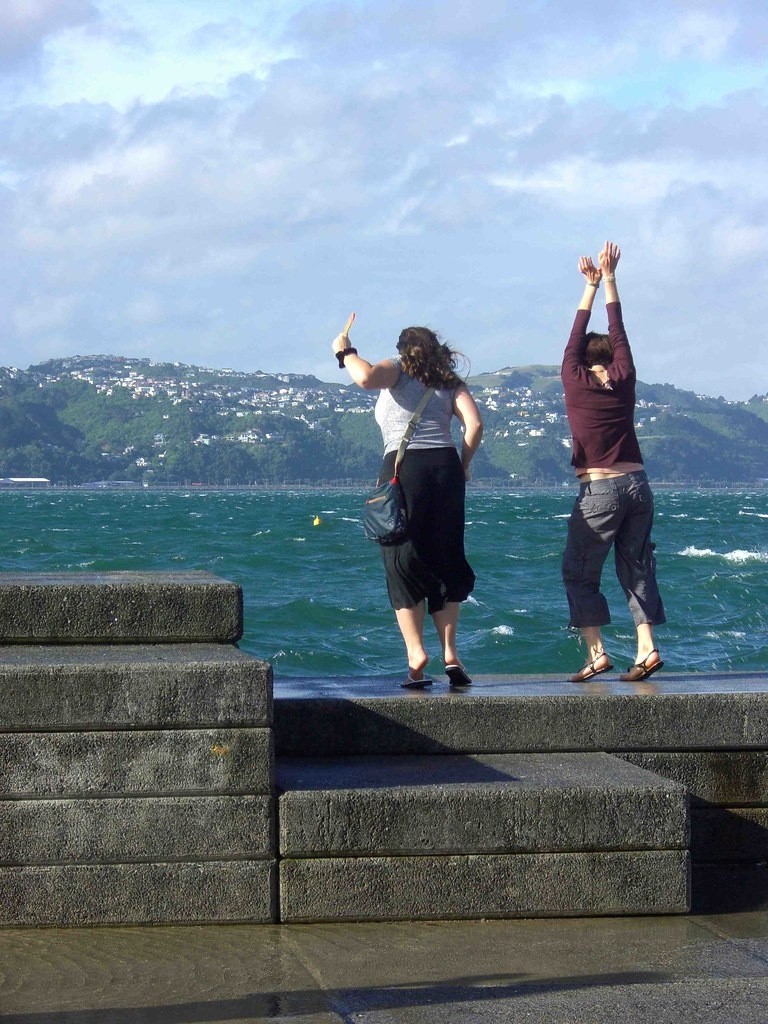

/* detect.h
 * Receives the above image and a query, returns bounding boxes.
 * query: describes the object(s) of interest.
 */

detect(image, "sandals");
[567,653,614,682]
[620,649,664,682]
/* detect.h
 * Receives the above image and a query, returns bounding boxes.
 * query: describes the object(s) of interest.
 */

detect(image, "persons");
[560,241,667,682]
[332,327,482,689]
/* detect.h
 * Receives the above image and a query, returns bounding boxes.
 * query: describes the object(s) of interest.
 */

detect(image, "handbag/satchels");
[361,478,410,544]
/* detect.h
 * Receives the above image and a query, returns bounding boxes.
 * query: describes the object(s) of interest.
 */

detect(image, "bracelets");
[334,346,357,368]
[585,279,599,288]
[602,277,616,282]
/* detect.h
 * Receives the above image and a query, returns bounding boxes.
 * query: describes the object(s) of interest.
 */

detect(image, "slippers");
[445,664,472,687]
[400,671,433,688]
